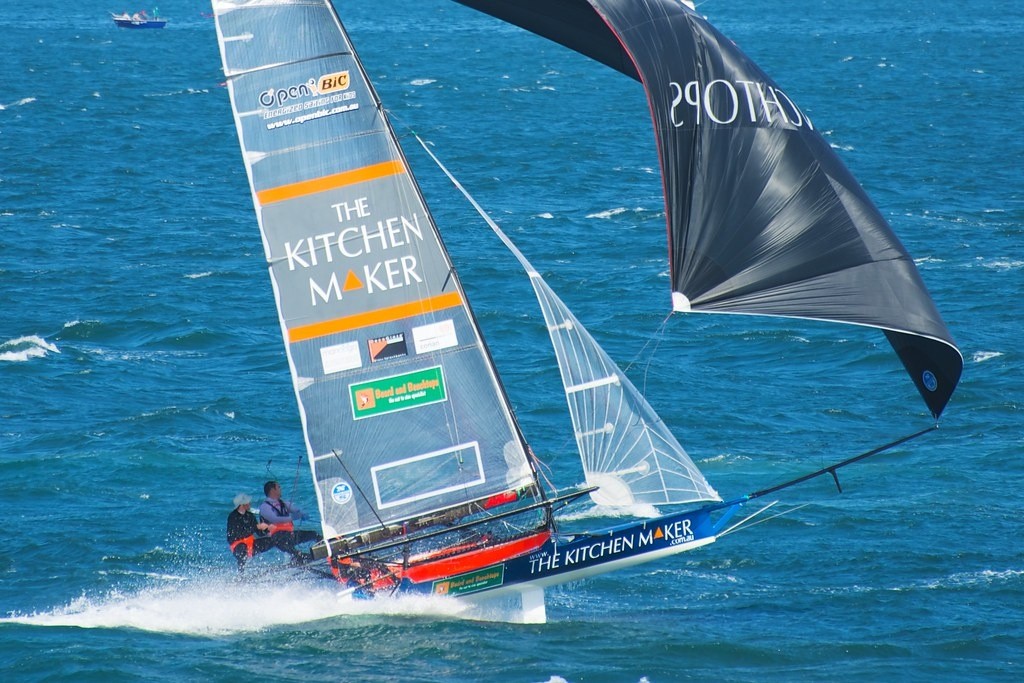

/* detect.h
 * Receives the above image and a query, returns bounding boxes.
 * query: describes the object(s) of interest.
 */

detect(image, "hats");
[233,493,253,510]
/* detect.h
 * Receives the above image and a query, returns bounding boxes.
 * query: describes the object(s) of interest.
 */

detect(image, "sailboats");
[208,0,964,608]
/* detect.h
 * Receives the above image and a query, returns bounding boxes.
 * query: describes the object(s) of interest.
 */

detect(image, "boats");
[111,7,167,29]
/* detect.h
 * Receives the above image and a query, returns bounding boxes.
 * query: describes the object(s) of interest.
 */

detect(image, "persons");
[123,7,160,21]
[226,481,325,570]
